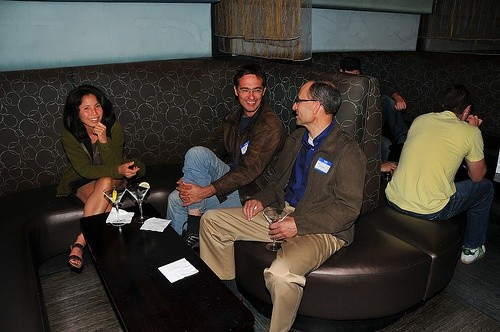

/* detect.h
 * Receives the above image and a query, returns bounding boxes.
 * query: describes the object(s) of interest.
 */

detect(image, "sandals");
[68,244,85,269]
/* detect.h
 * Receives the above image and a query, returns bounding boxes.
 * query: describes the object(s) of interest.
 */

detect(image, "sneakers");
[460,245,486,263]
[180,229,200,248]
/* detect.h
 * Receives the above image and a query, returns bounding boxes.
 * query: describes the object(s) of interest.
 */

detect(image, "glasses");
[237,88,263,95]
[295,96,318,104]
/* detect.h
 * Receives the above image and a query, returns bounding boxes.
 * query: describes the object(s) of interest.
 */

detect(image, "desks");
[81,203,256,332]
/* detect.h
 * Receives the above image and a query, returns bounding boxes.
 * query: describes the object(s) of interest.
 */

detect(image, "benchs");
[0,51,500,332]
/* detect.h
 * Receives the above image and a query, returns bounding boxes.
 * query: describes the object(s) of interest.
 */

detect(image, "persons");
[199,79,366,332]
[56,84,140,268]
[386,84,494,264]
[166,65,287,251]
[338,57,406,176]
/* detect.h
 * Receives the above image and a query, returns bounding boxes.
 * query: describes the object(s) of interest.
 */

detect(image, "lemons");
[138,182,150,189]
[113,190,117,201]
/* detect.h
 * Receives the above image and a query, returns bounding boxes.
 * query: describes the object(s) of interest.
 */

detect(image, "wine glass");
[126,182,150,223]
[263,209,289,252]
[102,184,127,226]
